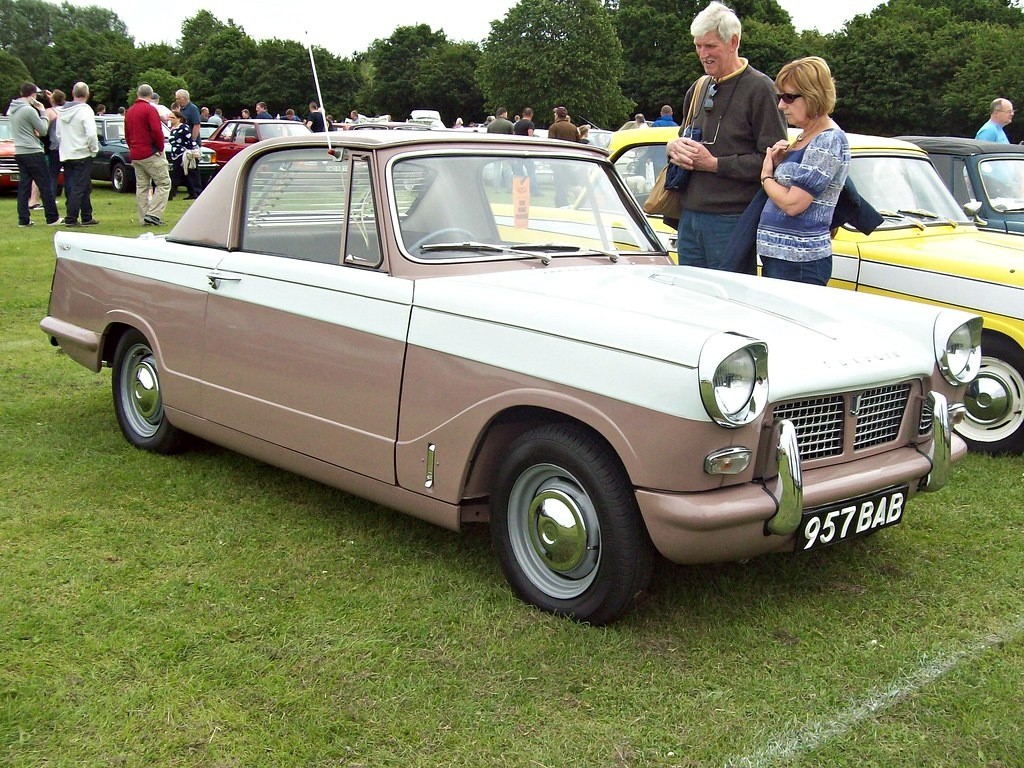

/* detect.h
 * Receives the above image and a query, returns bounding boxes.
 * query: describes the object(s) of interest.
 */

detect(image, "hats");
[152,94,161,99]
[553,107,567,116]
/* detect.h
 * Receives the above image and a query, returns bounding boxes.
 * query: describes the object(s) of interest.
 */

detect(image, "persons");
[236,102,274,119]
[96,104,106,116]
[486,116,495,121]
[649,105,678,175]
[199,107,209,122]
[514,107,545,196]
[279,109,301,122]
[9,82,99,227]
[755,57,849,286]
[124,84,172,226]
[579,124,594,145]
[347,110,359,129]
[975,97,1021,196]
[634,114,649,177]
[643,2,788,278]
[513,115,520,125]
[487,107,513,194]
[208,102,227,125]
[548,107,579,209]
[453,118,464,128]
[150,89,202,200]
[302,101,333,132]
[117,107,126,116]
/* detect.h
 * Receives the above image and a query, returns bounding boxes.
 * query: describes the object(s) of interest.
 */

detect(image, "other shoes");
[28,204,44,210]
[47,218,65,226]
[183,195,197,200]
[18,220,34,227]
[82,220,99,226]
[65,221,80,226]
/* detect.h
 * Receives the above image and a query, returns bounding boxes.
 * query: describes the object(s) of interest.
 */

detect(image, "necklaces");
[797,132,808,141]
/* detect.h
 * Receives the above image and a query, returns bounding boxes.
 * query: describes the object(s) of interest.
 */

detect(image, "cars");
[200,119,320,185]
[0,116,64,195]
[862,137,1024,233]
[489,126,1024,457]
[410,110,636,187]
[90,116,221,192]
[332,123,433,192]
[41,124,986,622]
[200,122,221,139]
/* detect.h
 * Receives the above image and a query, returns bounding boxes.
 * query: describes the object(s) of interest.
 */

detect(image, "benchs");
[244,228,432,269]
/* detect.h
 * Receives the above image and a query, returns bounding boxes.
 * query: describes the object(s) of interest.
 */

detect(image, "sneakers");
[144,215,166,226]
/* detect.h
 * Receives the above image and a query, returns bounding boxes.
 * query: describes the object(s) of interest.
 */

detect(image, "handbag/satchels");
[644,74,711,219]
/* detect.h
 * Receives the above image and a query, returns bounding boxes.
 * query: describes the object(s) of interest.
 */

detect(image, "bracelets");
[760,176,773,187]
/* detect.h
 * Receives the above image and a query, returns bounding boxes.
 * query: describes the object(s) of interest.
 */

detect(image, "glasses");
[997,110,1014,114]
[169,117,177,119]
[705,84,721,116]
[777,93,802,104]
